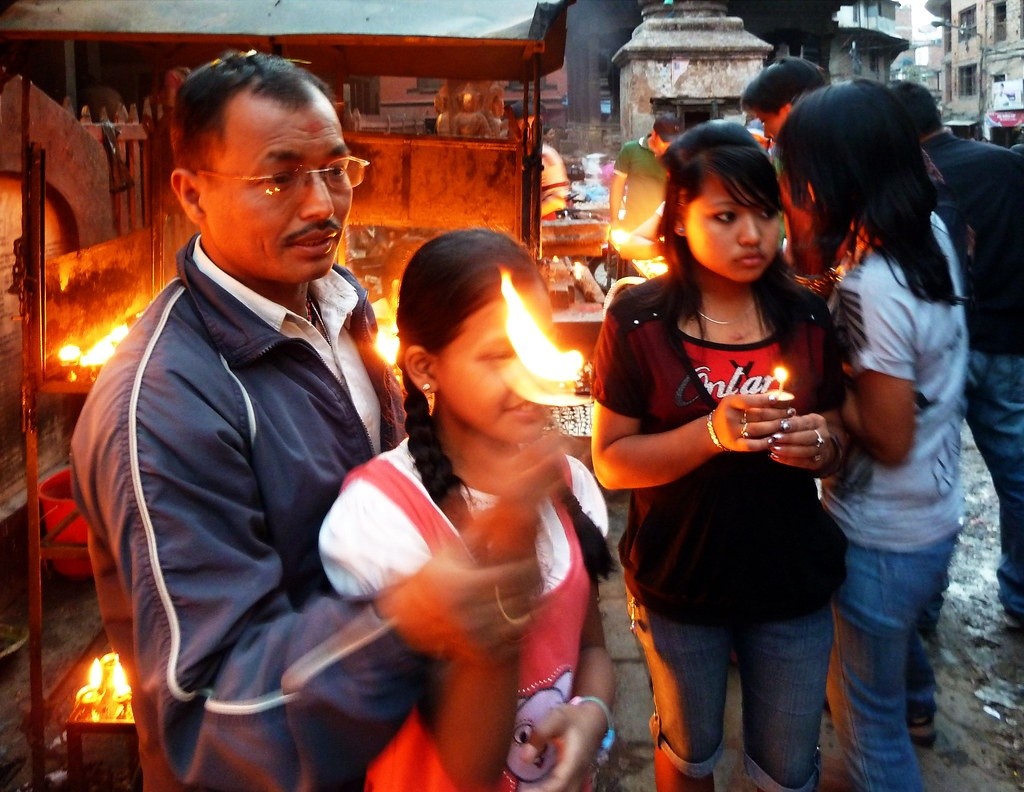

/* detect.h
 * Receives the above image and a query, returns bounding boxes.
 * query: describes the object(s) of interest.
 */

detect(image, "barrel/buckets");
[38,469,94,580]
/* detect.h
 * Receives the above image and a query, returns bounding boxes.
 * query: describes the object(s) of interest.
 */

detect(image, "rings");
[812,454,822,463]
[741,409,750,423]
[815,430,824,448]
[741,421,750,437]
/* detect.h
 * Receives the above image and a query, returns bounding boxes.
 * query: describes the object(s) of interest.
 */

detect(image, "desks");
[539,220,610,263]
[605,233,670,291]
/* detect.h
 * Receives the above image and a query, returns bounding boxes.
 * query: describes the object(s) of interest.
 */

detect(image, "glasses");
[197,156,370,202]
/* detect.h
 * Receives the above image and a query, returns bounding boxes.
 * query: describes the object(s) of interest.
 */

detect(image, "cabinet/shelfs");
[17,137,143,792]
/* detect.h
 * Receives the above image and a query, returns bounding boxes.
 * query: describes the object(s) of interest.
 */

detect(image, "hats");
[654,114,680,143]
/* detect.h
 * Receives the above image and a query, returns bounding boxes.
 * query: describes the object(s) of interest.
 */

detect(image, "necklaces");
[697,298,757,326]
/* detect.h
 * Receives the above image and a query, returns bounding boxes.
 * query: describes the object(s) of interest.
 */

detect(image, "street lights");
[933,18,990,144]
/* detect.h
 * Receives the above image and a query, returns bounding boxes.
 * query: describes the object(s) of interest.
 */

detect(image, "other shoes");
[907,719,936,746]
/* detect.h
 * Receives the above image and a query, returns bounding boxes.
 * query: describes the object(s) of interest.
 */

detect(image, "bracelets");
[707,409,733,452]
[828,430,845,464]
[492,583,539,625]
[569,696,619,771]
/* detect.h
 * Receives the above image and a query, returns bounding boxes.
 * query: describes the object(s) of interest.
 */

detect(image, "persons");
[61,48,543,792]
[543,126,571,204]
[741,55,855,269]
[745,118,778,156]
[885,74,1023,641]
[607,110,686,234]
[1009,130,1024,155]
[598,155,615,189]
[314,224,619,790]
[618,200,673,263]
[968,121,989,144]
[585,117,855,790]
[777,75,971,792]
